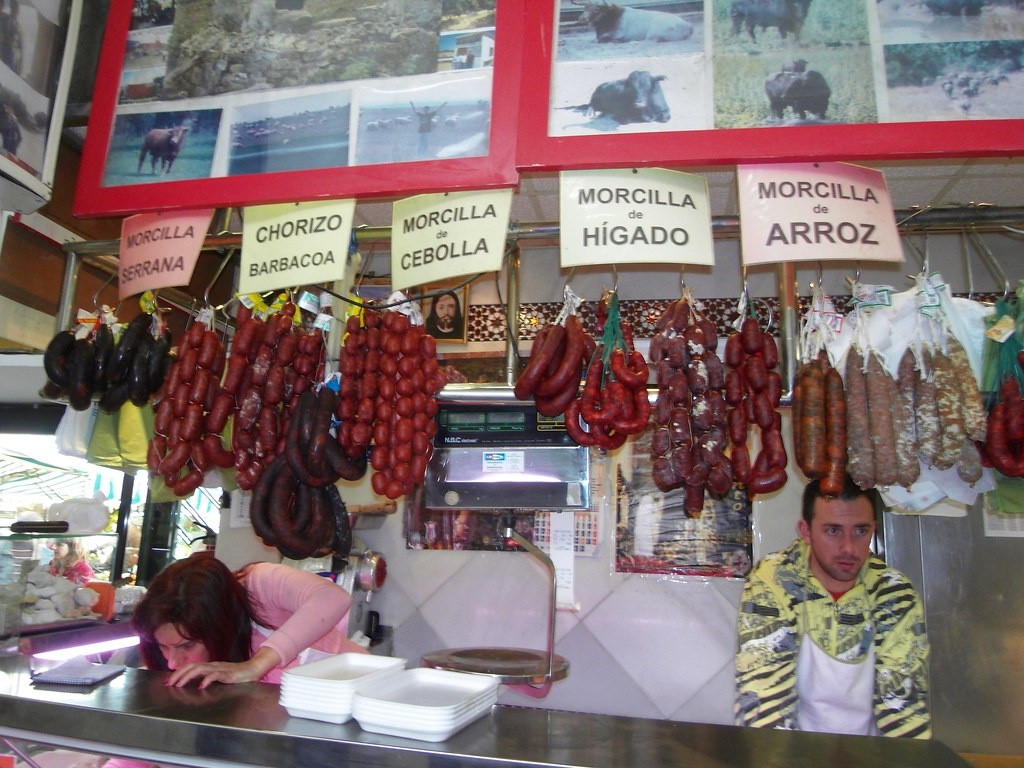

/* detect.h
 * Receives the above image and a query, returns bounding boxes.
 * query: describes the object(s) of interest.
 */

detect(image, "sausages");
[43,298,449,560]
[513,298,1024,520]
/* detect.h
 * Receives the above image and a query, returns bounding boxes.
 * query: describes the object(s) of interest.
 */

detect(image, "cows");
[569,0,832,125]
[135,124,192,175]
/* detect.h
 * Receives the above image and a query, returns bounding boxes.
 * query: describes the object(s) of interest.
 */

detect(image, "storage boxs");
[0,529,119,637]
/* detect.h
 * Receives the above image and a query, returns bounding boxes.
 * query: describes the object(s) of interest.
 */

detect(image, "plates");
[277,653,408,726]
[350,668,498,742]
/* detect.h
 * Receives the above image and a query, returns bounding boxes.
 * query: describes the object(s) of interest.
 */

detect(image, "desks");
[0,654,970,768]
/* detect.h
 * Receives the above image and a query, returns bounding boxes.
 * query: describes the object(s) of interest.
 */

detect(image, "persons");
[94,550,373,767]
[733,477,933,740]
[423,289,465,338]
[46,538,95,586]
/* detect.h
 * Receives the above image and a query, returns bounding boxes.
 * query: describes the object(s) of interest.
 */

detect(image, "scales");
[418,379,592,688]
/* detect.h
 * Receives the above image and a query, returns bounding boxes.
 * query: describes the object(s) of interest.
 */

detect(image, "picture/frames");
[515,0,1024,169]
[422,282,470,345]
[326,277,408,343]
[73,0,522,220]
